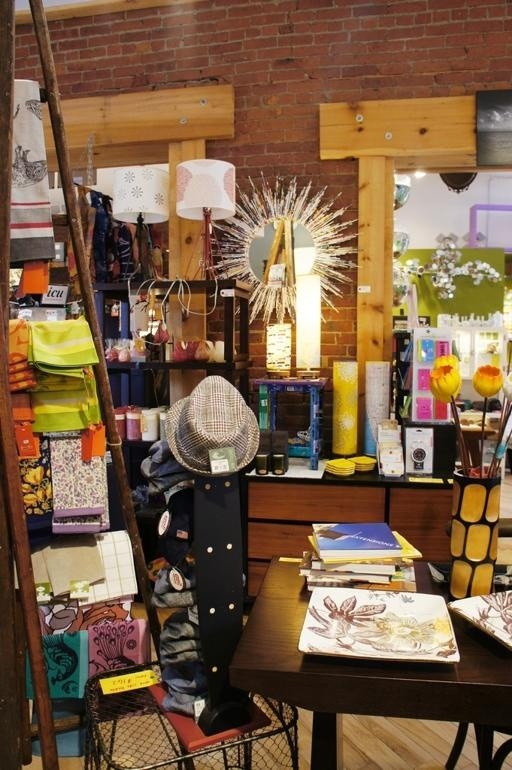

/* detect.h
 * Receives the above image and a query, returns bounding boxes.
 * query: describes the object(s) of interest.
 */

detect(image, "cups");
[113,405,169,443]
[272,454,285,476]
[256,454,269,475]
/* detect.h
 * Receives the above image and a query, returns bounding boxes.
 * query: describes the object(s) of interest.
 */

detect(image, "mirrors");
[210,173,363,325]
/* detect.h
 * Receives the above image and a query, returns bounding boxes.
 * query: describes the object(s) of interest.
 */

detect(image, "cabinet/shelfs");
[243,466,453,603]
[93,280,251,603]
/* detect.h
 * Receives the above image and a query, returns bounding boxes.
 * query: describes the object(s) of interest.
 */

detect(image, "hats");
[164,374,260,478]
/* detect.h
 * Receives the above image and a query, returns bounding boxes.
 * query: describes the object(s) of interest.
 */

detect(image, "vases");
[447,467,501,600]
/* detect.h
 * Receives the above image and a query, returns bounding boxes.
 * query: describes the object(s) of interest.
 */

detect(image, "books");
[296,522,424,595]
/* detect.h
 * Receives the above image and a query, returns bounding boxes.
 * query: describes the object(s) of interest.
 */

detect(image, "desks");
[226,554,512,770]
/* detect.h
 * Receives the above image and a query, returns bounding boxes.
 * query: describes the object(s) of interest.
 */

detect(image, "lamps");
[263,274,392,458]
[112,157,237,284]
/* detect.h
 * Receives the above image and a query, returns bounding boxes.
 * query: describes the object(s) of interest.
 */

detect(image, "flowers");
[429,338,512,479]
